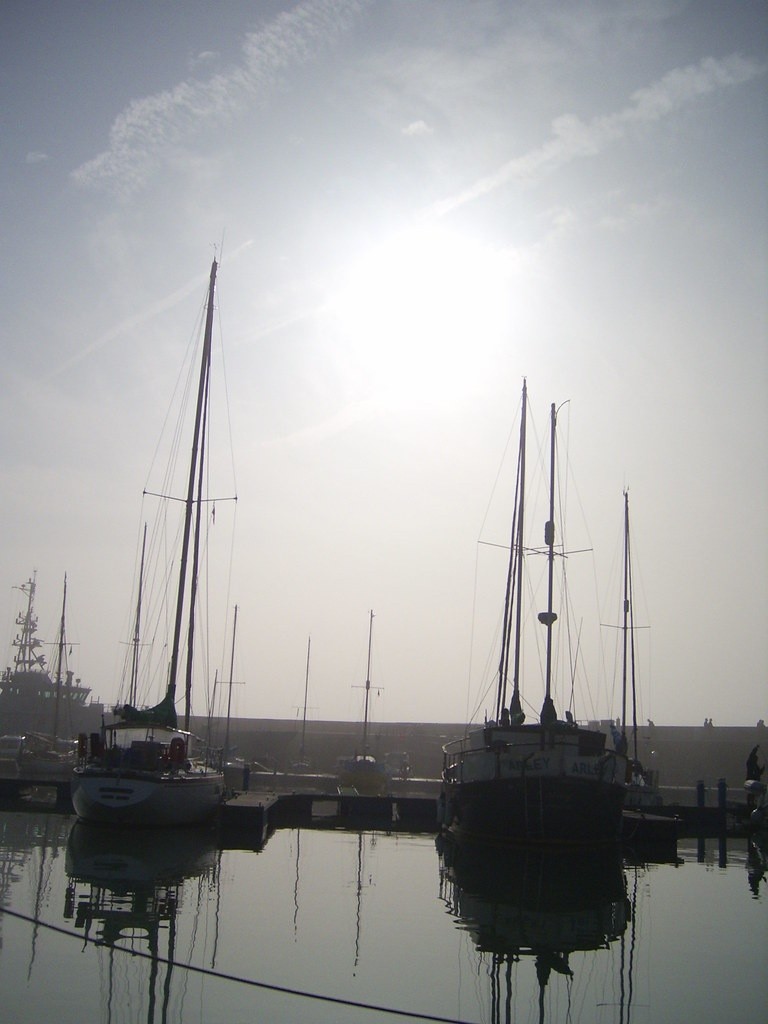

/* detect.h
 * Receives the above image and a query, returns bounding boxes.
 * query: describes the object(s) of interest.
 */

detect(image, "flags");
[610,725,625,753]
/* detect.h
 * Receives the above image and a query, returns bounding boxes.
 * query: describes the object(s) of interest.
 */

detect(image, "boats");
[624,808,682,839]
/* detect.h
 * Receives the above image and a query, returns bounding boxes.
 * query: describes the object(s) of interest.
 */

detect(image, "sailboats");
[13,572,76,775]
[439,377,632,847]
[70,258,226,830]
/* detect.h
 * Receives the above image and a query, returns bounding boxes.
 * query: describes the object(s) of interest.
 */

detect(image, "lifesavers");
[169,737,186,764]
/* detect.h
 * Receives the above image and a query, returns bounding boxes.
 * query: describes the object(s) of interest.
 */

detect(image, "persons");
[745,744,766,810]
[647,719,766,728]
